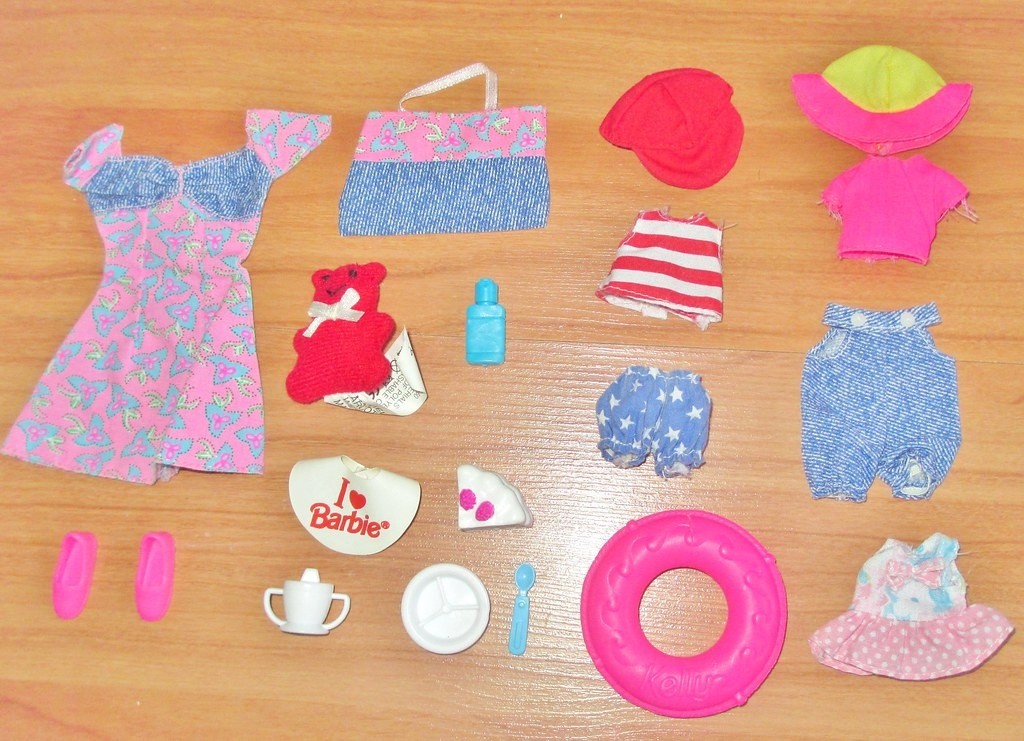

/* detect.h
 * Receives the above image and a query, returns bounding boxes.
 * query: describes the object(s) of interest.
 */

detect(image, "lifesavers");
[574,508,795,722]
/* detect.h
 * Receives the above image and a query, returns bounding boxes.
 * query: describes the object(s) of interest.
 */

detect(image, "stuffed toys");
[285,263,395,404]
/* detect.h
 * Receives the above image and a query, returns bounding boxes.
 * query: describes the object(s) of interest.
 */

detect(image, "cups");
[263,579,351,636]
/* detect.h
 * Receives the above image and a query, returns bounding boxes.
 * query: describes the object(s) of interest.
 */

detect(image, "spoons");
[508,563,536,656]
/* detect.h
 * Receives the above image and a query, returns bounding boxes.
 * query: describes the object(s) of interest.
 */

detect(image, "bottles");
[466,277,505,366]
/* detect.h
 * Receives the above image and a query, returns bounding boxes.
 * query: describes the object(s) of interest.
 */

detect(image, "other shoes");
[52,530,96,620]
[135,532,174,622]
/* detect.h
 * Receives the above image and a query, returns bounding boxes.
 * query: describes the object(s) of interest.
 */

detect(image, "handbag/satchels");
[340,62,550,236]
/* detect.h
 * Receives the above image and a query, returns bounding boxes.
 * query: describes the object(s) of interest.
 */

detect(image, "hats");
[793,45,974,157]
[599,68,743,190]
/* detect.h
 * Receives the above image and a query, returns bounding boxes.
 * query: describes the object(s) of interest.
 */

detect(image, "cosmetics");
[466,278,507,364]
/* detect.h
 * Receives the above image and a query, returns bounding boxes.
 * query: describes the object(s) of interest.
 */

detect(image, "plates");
[401,563,490,654]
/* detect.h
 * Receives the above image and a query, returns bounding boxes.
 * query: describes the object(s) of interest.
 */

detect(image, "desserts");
[457,464,533,531]
